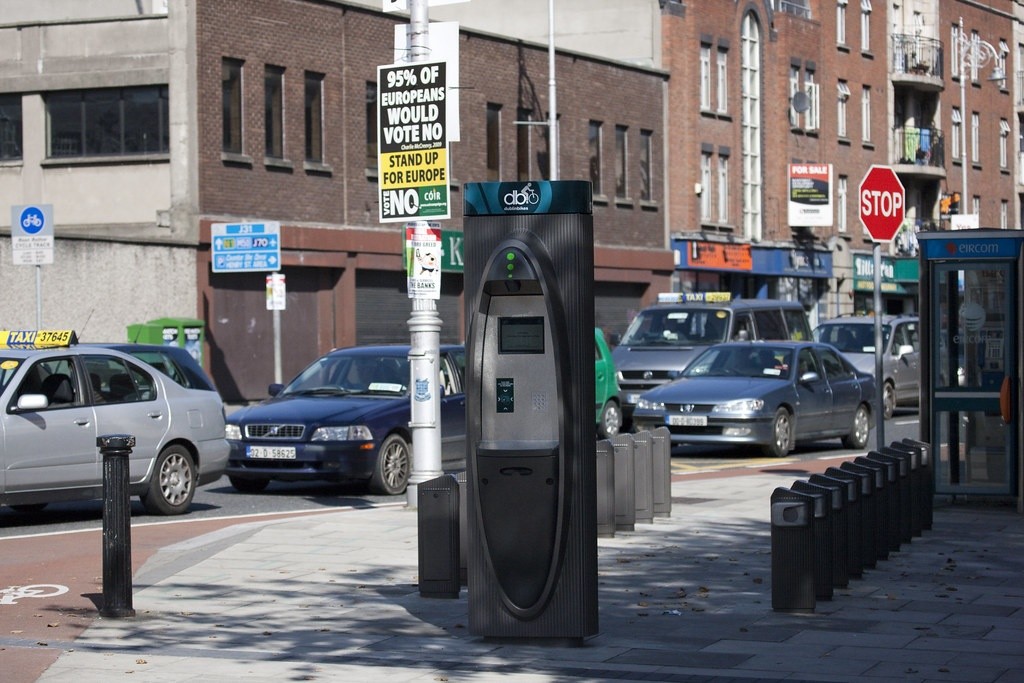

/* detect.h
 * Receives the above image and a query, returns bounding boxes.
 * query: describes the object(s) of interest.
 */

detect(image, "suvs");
[812,308,924,421]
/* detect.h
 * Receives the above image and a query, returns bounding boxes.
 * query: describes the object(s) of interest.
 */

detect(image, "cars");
[81,335,227,426]
[222,345,474,495]
[591,331,624,439]
[632,339,878,457]
[3,326,231,515]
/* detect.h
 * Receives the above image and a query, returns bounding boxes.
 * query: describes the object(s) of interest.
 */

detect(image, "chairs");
[756,350,780,374]
[838,328,856,351]
[398,363,410,387]
[89,373,102,394]
[107,374,140,402]
[39,374,74,406]
[375,359,399,383]
[784,353,804,380]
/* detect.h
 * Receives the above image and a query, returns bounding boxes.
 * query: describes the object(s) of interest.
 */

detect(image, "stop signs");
[860,163,909,245]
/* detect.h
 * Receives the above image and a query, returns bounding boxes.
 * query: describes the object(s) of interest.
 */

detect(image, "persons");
[830,328,849,349]
[655,316,688,341]
[707,317,738,342]
[773,353,807,381]
[355,359,375,390]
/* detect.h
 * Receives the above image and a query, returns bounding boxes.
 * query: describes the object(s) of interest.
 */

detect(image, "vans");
[607,291,815,433]
